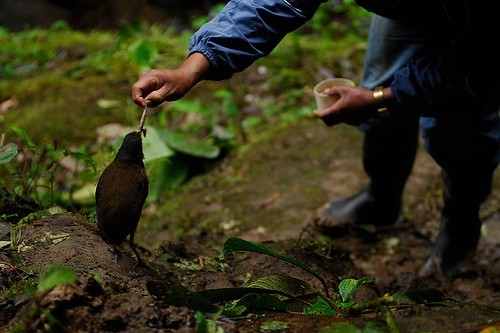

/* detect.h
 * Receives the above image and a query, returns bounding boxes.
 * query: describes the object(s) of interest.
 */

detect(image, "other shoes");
[312,188,402,234]
[410,232,478,291]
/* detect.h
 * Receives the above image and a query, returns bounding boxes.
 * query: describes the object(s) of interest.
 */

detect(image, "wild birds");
[95,130,151,270]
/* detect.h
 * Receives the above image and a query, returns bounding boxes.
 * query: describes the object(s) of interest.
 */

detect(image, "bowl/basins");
[313,78,355,111]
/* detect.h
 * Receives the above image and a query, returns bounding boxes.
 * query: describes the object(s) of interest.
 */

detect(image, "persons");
[130,0,499,288]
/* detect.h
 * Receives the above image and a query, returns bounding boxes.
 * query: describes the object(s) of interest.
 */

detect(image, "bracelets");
[374,86,391,117]
